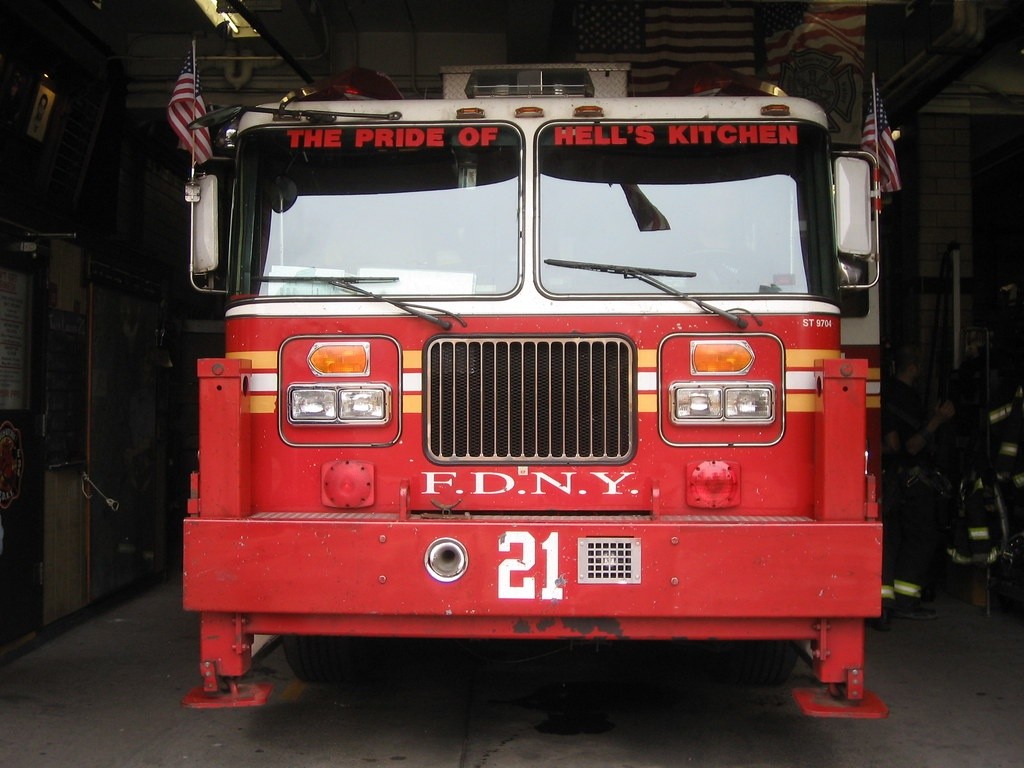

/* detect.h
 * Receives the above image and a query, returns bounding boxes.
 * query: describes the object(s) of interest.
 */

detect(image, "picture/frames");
[23,76,57,144]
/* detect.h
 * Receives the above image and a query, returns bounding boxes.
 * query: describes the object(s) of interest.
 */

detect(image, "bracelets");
[921,427,931,442]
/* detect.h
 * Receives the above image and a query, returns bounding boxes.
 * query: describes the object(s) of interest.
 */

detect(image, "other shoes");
[894,597,938,621]
[867,607,892,631]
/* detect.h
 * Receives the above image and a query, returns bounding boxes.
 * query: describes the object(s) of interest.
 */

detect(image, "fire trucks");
[171,33,900,724]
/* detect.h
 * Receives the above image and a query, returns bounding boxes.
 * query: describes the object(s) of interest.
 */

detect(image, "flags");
[166,49,214,165]
[574,0,866,146]
[861,84,902,192]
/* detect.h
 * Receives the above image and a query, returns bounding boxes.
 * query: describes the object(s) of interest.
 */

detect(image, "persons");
[3,70,23,122]
[30,95,49,133]
[872,342,955,630]
